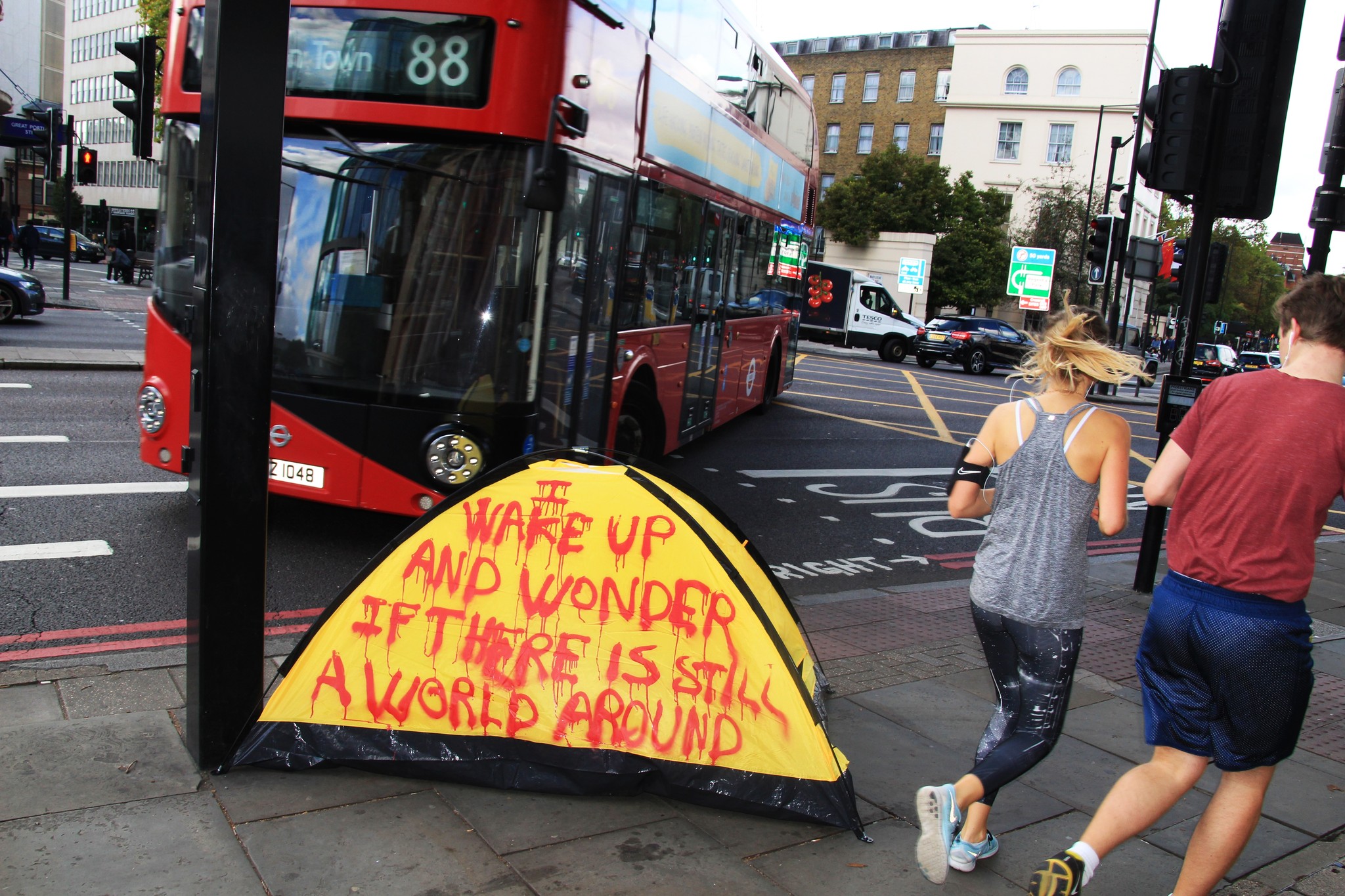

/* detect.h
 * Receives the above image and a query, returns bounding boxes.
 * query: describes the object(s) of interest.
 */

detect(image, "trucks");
[800,258,927,364]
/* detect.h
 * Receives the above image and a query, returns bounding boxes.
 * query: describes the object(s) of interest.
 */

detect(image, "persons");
[100,244,132,284]
[0,211,17,267]
[1140,331,1176,363]
[910,299,1151,886]
[1025,272,1345,896]
[860,290,873,309]
[17,220,40,269]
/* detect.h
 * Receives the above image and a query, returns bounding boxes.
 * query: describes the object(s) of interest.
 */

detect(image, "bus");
[138,1,822,517]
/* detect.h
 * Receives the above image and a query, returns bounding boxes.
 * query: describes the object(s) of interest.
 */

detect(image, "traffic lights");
[1166,235,1192,296]
[77,149,98,183]
[693,247,698,263]
[1086,213,1115,286]
[576,232,582,237]
[32,108,61,181]
[706,245,712,264]
[1133,63,1215,193]
[112,31,156,159]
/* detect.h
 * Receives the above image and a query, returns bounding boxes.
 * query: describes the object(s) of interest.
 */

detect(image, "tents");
[215,446,873,843]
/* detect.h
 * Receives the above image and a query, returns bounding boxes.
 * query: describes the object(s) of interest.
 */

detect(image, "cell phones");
[946,443,970,496]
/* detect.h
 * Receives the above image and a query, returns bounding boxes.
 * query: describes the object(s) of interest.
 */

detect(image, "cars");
[12,225,107,262]
[558,248,798,319]
[0,264,46,324]
[1192,343,1284,387]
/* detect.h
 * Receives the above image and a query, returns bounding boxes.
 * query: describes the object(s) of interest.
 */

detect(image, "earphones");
[1288,331,1294,346]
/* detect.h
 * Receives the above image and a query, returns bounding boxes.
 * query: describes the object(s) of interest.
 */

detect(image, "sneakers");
[1027,849,1085,896]
[916,783,962,885]
[948,826,998,871]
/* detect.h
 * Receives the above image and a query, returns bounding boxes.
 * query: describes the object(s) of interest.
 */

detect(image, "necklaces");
[1044,389,1085,397]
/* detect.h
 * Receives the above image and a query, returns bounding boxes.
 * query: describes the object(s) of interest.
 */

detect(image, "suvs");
[1103,319,1160,387]
[911,315,1040,378]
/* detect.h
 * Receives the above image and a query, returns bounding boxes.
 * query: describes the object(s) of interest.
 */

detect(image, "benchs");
[119,258,155,287]
